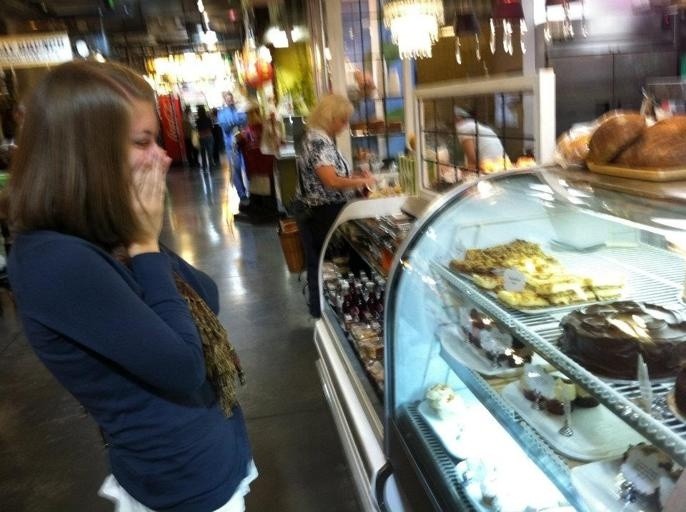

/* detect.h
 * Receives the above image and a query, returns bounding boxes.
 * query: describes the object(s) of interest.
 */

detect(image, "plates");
[667,388,686,427]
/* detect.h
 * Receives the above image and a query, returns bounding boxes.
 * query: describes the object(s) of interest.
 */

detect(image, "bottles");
[323,270,387,325]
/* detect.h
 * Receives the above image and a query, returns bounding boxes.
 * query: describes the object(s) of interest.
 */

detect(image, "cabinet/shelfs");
[378,162,686,512]
[305,185,432,512]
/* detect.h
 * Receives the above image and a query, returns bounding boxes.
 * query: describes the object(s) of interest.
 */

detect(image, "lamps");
[380,0,445,63]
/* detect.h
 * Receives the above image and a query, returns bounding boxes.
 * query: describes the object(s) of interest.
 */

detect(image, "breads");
[587,114,645,164]
[619,117,686,170]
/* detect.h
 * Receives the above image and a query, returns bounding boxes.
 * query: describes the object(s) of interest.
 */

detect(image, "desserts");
[460,308,534,368]
[621,442,686,512]
[521,363,600,414]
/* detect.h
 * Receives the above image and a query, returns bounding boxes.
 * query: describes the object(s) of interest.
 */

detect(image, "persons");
[5,60,260,512]
[181,89,247,200]
[453,102,513,176]
[294,95,380,317]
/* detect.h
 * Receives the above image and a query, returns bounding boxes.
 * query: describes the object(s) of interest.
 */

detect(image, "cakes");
[557,300,686,381]
[667,367,686,425]
